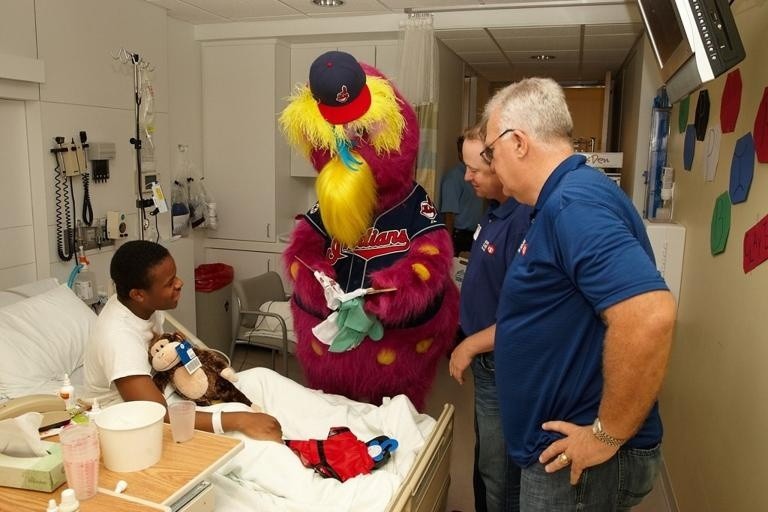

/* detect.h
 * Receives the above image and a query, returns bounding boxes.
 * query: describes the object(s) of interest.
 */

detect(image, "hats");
[308,51,372,125]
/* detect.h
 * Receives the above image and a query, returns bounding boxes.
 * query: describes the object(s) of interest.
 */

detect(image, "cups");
[59,421,102,503]
[167,399,195,445]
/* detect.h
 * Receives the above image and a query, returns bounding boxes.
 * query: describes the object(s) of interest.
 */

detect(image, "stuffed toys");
[148,332,258,410]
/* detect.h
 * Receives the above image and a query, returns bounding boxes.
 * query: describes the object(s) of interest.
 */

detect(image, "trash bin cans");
[194,263,234,360]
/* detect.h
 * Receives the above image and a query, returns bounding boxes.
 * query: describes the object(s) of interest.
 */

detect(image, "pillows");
[0,283,97,398]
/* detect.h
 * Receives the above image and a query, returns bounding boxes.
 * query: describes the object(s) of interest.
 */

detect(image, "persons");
[478,78,677,512]
[83,240,284,445]
[440,133,485,261]
[449,125,536,512]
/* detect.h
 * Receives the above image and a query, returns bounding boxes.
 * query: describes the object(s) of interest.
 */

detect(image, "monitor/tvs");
[637,0,747,106]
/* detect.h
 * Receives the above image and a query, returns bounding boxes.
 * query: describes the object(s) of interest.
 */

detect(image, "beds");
[0,276,453,512]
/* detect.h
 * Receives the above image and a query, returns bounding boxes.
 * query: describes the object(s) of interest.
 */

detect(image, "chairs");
[228,271,298,377]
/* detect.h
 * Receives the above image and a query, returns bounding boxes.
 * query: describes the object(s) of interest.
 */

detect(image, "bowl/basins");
[92,399,168,473]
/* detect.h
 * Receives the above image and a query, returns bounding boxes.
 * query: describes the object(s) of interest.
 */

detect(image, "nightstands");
[77,234,197,333]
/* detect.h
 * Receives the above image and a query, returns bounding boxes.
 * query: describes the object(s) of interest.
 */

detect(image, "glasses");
[479,128,528,165]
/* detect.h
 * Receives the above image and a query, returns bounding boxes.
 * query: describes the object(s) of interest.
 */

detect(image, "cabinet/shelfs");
[190,13,404,356]
